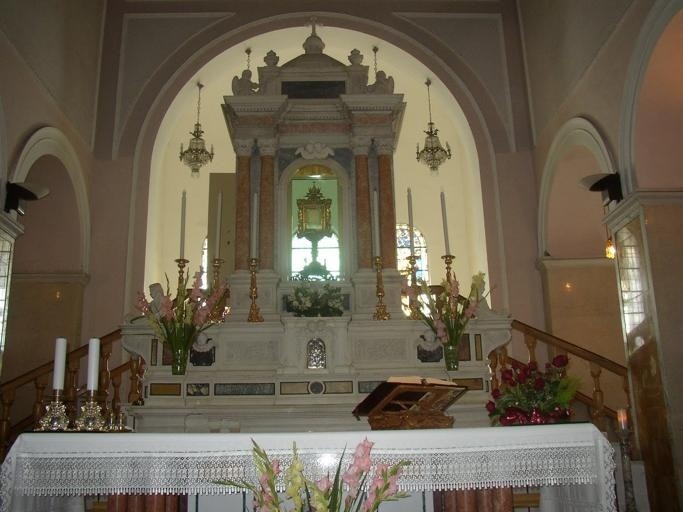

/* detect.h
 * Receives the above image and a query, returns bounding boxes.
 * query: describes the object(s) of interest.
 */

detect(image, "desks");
[14,422,609,512]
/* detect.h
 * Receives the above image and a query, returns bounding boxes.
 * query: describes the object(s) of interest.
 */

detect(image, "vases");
[444,347,459,371]
[172,348,189,375]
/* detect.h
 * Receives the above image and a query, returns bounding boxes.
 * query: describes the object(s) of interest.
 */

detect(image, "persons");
[149,283,171,314]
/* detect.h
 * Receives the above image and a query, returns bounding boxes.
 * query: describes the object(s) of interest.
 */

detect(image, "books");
[351,376,468,417]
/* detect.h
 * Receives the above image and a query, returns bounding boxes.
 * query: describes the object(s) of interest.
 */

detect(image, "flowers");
[402,272,497,369]
[212,437,413,511]
[484,355,585,424]
[282,279,344,316]
[130,265,232,372]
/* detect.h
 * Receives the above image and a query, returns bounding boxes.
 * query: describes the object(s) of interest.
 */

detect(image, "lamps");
[178,82,216,179]
[413,78,452,172]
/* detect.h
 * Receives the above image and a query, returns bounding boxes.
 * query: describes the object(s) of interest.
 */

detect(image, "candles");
[86,339,101,391]
[372,190,450,256]
[53,338,66,390]
[179,189,260,261]
